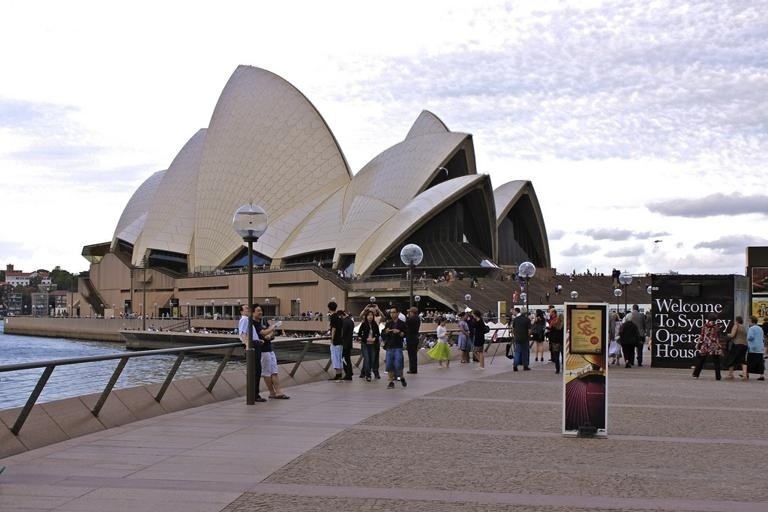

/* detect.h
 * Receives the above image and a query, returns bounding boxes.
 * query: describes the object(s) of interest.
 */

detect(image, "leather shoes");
[256,398,267,402]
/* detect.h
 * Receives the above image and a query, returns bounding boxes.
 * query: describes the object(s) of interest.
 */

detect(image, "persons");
[238,304,267,402]
[328,262,652,388]
[746,315,765,380]
[690,314,723,379]
[54,310,324,340]
[724,316,748,380]
[252,304,290,399]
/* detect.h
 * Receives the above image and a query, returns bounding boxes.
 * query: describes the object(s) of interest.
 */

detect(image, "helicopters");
[653,239,662,243]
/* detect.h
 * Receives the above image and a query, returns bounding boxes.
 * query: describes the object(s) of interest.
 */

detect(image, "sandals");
[268,395,275,399]
[274,393,290,400]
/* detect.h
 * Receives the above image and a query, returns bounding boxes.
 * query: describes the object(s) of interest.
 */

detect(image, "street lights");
[140,303,143,316]
[203,303,207,318]
[237,299,241,318]
[88,304,92,318]
[154,302,157,318]
[518,261,536,318]
[232,194,270,407]
[297,298,301,318]
[113,304,116,318]
[222,302,227,318]
[265,299,270,317]
[369,296,376,309]
[211,301,215,318]
[465,295,473,309]
[612,288,622,314]
[618,269,632,315]
[186,302,190,318]
[398,243,423,309]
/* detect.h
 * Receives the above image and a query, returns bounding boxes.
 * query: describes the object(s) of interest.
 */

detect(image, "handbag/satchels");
[484,326,490,335]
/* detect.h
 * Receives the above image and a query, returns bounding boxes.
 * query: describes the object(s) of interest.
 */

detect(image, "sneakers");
[367,377,371,382]
[387,381,394,389]
[400,376,407,387]
